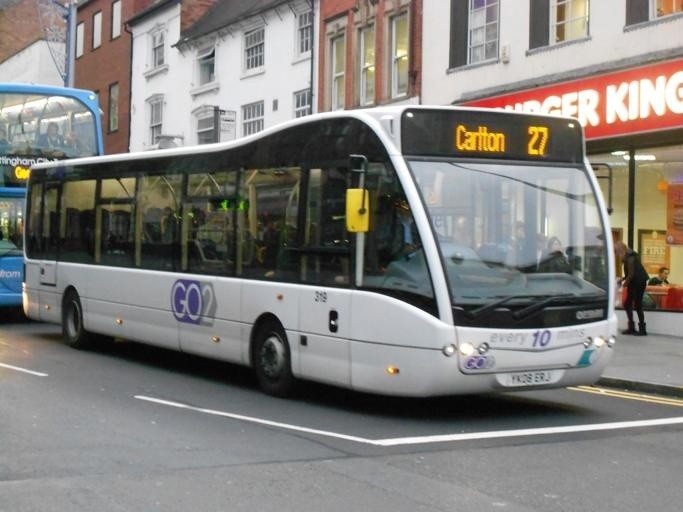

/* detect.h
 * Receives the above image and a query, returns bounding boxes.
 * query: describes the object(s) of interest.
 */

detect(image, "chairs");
[47,206,320,283]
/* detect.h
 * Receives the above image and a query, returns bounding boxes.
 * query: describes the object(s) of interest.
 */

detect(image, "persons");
[387,192,459,263]
[613,241,650,336]
[450,215,584,281]
[157,204,298,279]
[33,120,73,157]
[0,125,18,156]
[646,267,670,285]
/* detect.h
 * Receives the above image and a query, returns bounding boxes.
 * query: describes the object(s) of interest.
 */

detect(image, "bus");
[0,82,105,310]
[21,103,618,398]
